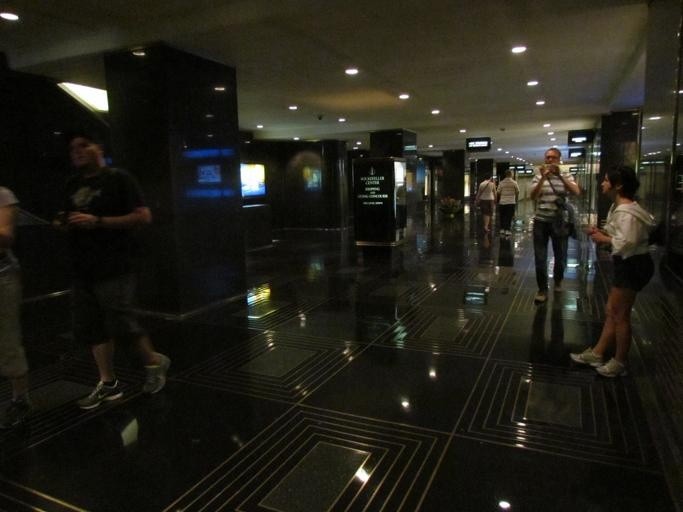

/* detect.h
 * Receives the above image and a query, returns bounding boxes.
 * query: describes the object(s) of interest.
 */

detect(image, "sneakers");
[498,225,514,237]
[140,353,172,398]
[480,224,492,234]
[594,356,632,380]
[77,378,124,412]
[568,346,604,368]
[1,392,35,431]
[533,288,548,306]
[552,280,564,294]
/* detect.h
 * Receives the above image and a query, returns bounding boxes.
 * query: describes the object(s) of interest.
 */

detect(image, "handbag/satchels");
[554,203,576,240]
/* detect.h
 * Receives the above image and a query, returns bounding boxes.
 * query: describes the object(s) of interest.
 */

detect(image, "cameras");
[541,163,553,169]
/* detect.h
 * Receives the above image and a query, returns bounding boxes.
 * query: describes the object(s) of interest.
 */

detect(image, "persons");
[496,171,520,236]
[0,187,34,423]
[473,170,495,231]
[474,231,492,293]
[526,292,567,429]
[570,158,659,377]
[53,129,172,410]
[490,233,515,295]
[529,147,581,304]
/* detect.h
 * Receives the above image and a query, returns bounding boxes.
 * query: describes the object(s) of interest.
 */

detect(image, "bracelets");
[92,215,102,229]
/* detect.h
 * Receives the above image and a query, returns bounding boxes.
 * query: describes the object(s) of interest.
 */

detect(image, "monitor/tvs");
[239,163,267,199]
